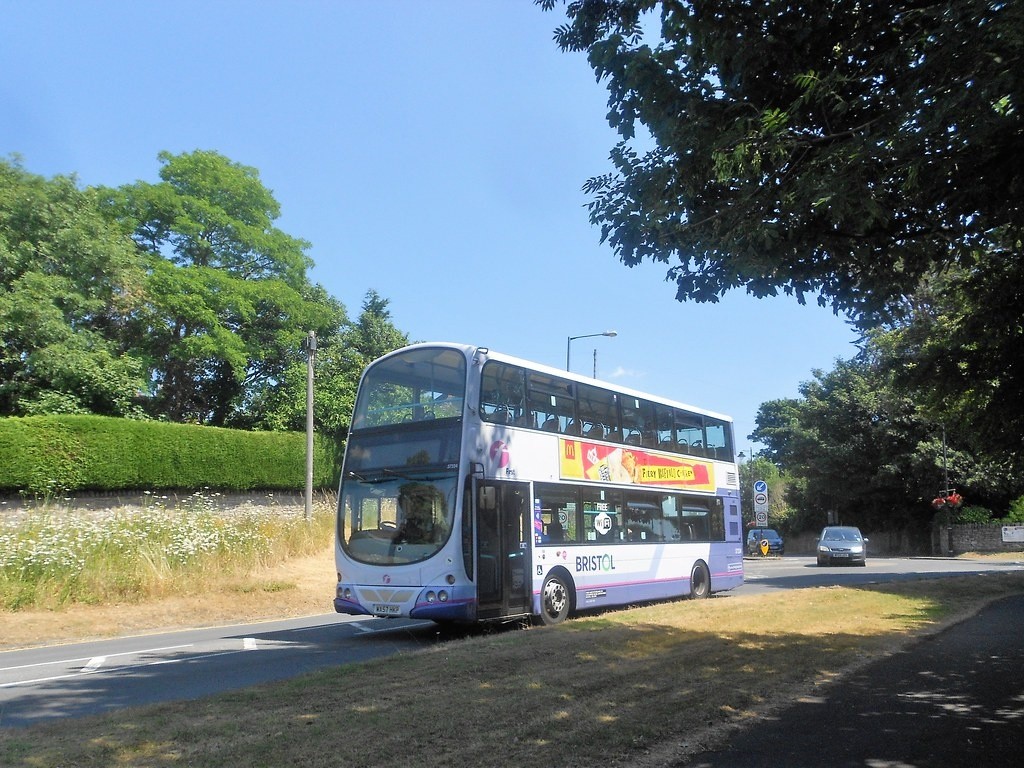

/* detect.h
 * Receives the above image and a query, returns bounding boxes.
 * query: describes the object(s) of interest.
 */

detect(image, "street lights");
[737,446,754,520]
[567,329,619,372]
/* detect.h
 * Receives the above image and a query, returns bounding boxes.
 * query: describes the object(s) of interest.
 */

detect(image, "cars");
[747,529,784,556]
[814,526,870,567]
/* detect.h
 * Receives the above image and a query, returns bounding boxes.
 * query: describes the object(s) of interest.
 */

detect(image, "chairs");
[401,411,436,422]
[480,403,717,459]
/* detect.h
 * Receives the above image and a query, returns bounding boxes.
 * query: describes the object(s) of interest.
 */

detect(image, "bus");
[331,342,743,632]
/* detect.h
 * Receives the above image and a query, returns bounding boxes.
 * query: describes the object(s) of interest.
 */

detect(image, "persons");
[396,493,433,544]
